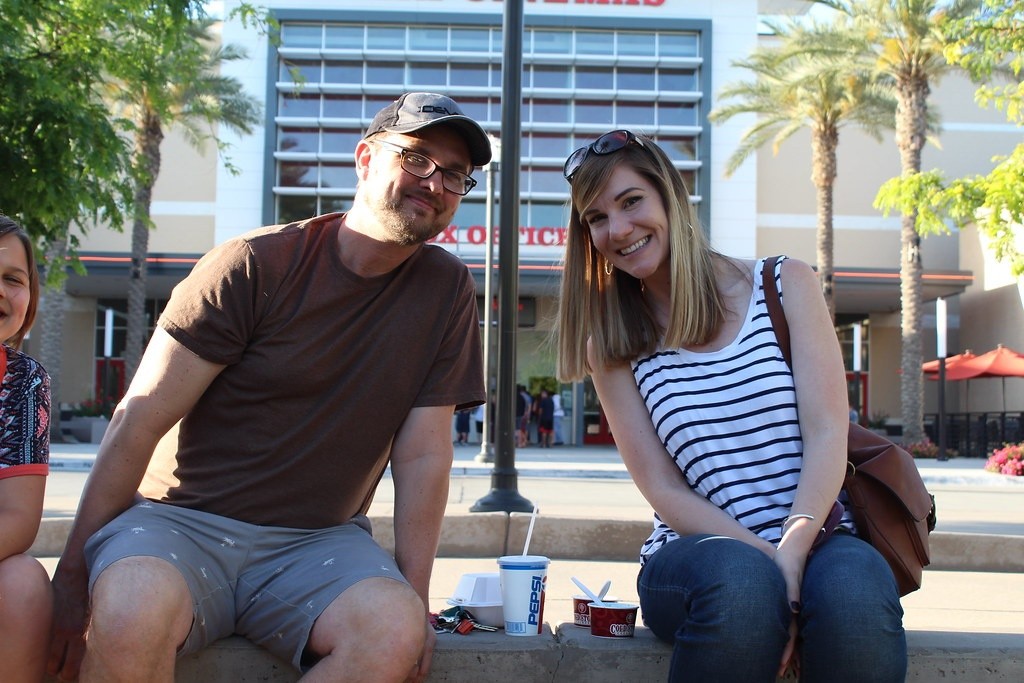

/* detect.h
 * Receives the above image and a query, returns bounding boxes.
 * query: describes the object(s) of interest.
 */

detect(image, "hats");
[362,91,492,167]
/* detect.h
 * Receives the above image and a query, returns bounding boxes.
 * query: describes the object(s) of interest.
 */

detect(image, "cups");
[586,602,639,640]
[572,595,617,628]
[497,555,551,637]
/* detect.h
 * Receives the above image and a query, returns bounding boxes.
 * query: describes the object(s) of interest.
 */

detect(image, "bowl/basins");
[446,572,505,628]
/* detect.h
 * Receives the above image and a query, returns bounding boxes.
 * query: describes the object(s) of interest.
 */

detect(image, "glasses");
[561,128,650,187]
[371,137,477,196]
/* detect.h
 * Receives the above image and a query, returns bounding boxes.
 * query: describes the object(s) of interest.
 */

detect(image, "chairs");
[923,412,1024,459]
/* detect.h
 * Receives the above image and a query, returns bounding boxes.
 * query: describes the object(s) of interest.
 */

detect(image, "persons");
[47,91,486,682]
[556,129,910,683]
[0,213,55,683]
[457,385,557,447]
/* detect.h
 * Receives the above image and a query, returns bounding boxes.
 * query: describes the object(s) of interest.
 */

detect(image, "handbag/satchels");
[842,417,934,598]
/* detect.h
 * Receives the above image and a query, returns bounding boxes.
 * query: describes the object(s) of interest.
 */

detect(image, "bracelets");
[781,514,827,554]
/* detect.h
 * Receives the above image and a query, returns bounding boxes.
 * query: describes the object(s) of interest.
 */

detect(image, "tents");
[917,344,1022,458]
